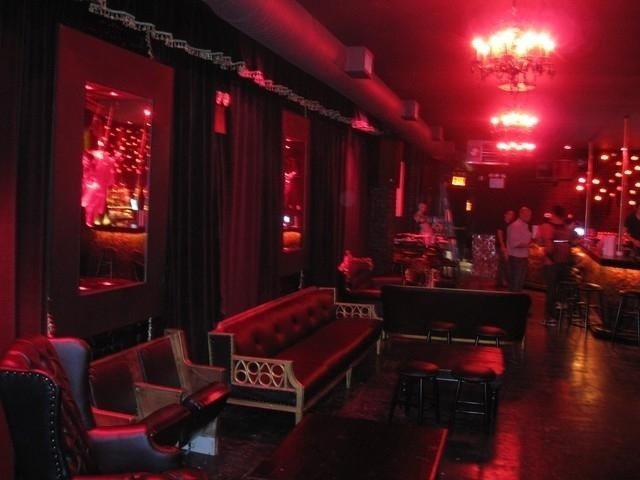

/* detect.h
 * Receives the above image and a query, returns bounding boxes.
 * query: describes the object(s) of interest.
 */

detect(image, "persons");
[131,183,144,210]
[412,202,433,235]
[138,187,150,215]
[495,200,640,328]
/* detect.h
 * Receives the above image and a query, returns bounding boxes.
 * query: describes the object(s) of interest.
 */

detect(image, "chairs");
[3,328,233,480]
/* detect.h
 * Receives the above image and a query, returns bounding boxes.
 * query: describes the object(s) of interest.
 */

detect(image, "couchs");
[380,282,531,352]
[206,287,384,425]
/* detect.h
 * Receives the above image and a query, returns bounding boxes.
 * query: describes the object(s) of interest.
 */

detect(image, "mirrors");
[281,108,311,255]
[78,81,153,296]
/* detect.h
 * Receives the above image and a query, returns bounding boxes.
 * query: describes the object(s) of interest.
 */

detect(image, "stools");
[96,246,118,279]
[389,321,505,464]
[555,267,640,348]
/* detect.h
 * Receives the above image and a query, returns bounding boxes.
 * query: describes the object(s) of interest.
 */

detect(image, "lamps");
[468,1,556,92]
[212,90,230,133]
